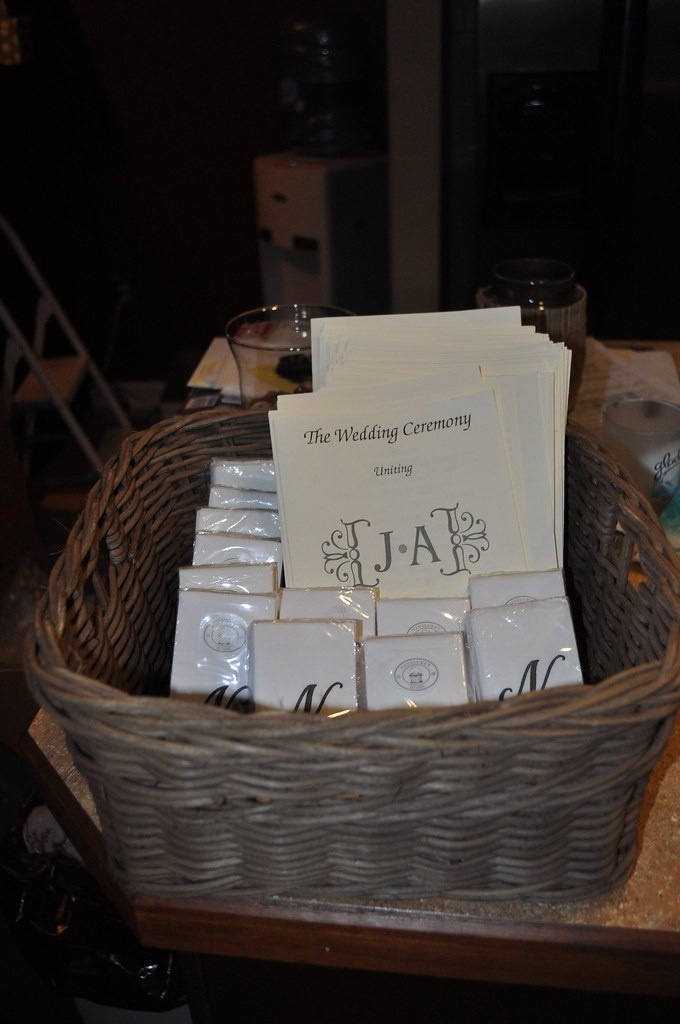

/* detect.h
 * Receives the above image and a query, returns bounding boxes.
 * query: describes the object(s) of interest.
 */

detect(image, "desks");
[17,338,680,996]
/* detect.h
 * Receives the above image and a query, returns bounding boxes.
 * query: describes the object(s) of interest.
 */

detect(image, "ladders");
[0,216,133,511]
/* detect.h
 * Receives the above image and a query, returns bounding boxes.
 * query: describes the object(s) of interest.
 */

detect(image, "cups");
[225,301,357,411]
[599,397,679,553]
[474,256,588,412]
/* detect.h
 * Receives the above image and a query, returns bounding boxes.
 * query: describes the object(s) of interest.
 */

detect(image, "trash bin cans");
[13,862,192,1024]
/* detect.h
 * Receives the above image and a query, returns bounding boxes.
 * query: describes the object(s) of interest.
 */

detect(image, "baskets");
[22,397,680,902]
